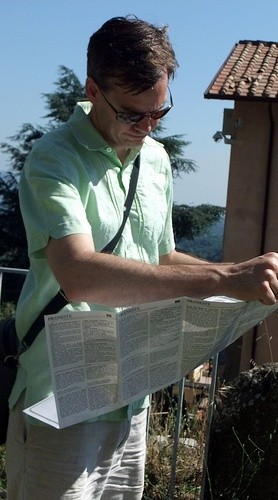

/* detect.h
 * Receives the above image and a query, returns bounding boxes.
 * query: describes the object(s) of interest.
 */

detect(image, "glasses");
[92,79,174,124]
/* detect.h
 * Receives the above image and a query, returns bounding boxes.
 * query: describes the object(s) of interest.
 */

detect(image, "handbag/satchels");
[0,317,23,447]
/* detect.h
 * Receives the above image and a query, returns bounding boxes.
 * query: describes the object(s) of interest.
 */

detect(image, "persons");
[4,14,278,500]
